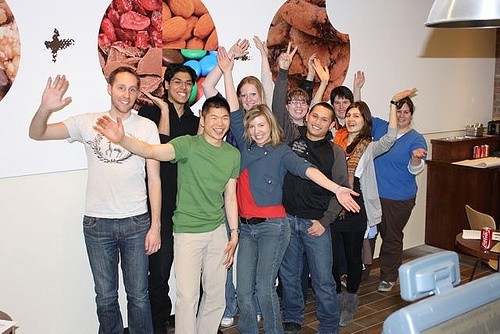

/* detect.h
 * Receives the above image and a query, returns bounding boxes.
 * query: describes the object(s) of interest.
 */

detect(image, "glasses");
[286,101,307,106]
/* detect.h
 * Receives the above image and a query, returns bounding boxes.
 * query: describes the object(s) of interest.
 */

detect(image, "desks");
[425,133,500,257]
[454,232,500,282]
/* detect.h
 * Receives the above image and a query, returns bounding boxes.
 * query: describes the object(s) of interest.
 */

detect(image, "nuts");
[162,0,219,50]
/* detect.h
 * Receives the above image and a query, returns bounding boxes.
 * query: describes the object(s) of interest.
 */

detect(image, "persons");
[216,45,361,334]
[199,38,428,334]
[138,63,203,334]
[28,66,161,334]
[93,96,241,334]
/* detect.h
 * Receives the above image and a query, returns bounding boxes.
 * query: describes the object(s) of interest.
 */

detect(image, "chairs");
[465,204,500,283]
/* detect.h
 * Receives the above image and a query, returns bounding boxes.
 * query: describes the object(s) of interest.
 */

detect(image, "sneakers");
[378,281,395,291]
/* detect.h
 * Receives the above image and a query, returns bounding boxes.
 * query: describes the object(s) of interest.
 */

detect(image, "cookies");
[267,0,351,105]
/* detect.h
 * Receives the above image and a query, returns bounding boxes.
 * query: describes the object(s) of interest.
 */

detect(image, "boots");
[335,288,359,326]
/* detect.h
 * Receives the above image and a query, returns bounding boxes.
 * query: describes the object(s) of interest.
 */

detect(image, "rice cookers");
[488,120,500,134]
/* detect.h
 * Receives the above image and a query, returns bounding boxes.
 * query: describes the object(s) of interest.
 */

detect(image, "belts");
[238,217,270,226]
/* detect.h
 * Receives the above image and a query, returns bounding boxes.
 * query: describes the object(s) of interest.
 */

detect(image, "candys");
[162,48,218,107]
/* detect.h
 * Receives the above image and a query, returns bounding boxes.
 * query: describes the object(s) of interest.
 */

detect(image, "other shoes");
[281,322,301,334]
[361,264,369,283]
[217,329,223,334]
[257,315,261,322]
[221,318,233,327]
[341,275,347,286]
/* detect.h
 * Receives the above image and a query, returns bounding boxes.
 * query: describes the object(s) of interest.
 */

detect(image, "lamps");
[424,0,500,29]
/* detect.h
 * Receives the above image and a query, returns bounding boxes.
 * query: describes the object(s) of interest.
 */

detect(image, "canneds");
[480,226,492,249]
[473,144,489,158]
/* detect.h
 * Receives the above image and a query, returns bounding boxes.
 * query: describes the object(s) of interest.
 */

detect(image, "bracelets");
[390,100,400,105]
